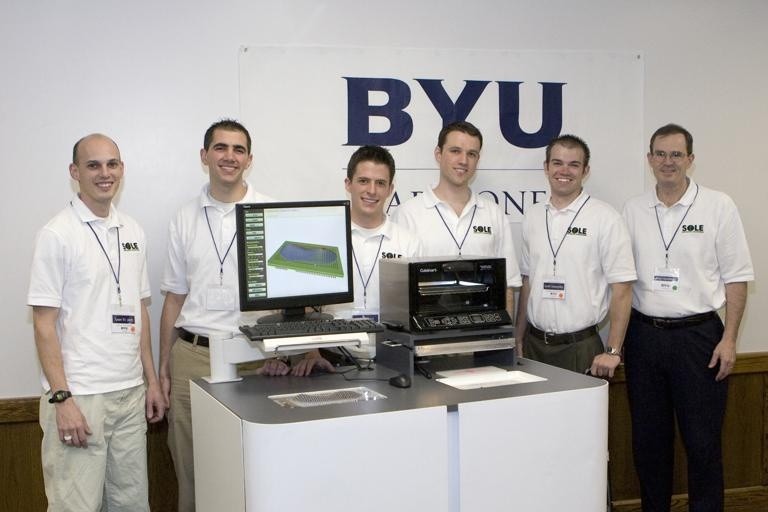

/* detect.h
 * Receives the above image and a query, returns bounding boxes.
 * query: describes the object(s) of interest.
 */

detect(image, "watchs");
[604,346,622,357]
[49,389,72,407]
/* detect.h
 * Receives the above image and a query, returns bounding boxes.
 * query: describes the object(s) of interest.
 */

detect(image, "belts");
[177,327,210,347]
[631,306,716,331]
[530,324,596,344]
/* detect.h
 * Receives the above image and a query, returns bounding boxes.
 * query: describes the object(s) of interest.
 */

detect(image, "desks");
[189,352,610,510]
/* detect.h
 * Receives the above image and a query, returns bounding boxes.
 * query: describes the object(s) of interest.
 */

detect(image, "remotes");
[382,321,404,330]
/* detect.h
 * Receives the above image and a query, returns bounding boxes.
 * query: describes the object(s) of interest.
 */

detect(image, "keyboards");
[239,320,388,340]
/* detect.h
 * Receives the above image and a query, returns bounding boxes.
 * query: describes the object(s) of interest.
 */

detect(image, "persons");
[290,144,425,377]
[390,121,524,324]
[25,133,165,512]
[158,118,291,512]
[515,134,638,380]
[619,122,755,512]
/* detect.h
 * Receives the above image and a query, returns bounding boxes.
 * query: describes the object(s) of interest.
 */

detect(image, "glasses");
[653,152,688,161]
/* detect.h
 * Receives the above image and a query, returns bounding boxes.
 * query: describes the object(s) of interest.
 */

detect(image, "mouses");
[389,375,413,388]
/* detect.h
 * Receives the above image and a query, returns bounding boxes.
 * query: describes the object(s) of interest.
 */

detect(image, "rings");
[65,435,72,443]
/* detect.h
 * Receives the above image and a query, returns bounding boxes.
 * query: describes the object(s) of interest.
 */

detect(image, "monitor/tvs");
[235,199,354,324]
